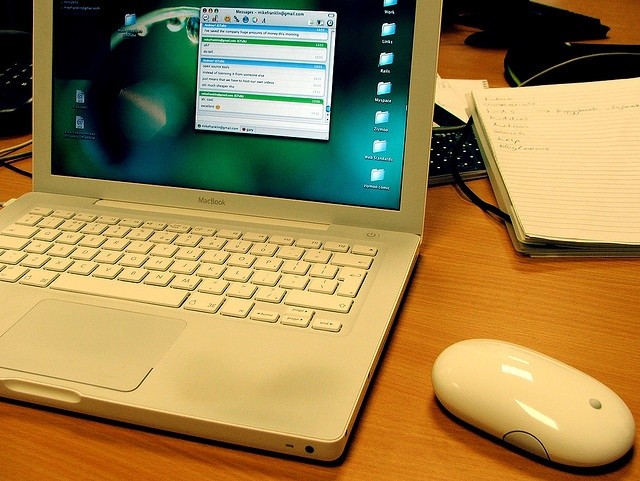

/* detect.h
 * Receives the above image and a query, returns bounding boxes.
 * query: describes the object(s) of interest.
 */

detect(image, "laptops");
[0,0,444,464]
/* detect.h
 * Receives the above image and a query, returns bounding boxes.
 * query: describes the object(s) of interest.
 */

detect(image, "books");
[465,75,640,261]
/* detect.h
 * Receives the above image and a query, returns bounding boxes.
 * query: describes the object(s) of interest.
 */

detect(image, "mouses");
[431,337,636,469]
